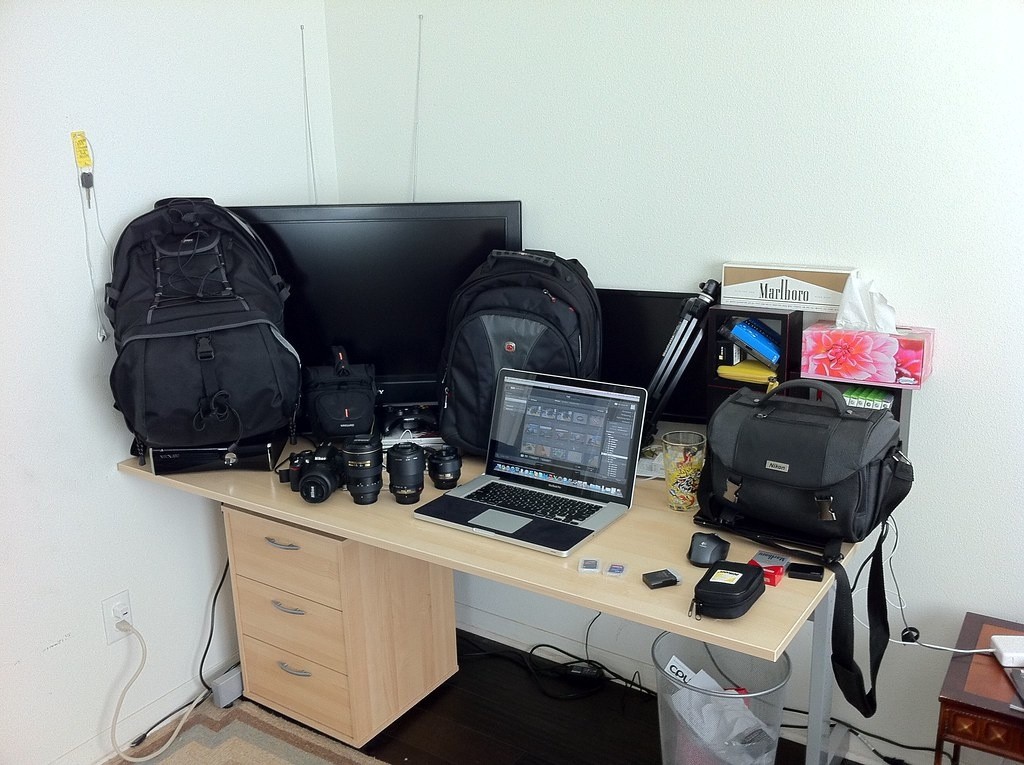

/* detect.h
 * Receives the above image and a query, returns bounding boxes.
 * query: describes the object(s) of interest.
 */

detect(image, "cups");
[662,430,707,513]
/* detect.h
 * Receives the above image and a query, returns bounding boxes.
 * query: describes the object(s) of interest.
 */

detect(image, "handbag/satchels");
[303,345,378,441]
[696,376,915,545]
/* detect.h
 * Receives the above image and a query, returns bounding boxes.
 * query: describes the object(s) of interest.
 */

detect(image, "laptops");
[413,367,648,557]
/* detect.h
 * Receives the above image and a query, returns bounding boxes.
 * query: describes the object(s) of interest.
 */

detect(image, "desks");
[115,434,864,765]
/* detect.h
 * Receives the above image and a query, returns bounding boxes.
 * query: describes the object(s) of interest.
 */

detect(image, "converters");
[568,664,603,684]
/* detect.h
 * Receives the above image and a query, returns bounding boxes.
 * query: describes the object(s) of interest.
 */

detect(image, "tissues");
[801,268,938,391]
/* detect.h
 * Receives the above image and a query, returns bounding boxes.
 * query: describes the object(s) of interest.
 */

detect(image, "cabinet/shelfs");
[219,502,461,749]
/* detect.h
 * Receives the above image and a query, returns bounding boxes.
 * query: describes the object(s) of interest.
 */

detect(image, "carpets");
[100,695,392,765]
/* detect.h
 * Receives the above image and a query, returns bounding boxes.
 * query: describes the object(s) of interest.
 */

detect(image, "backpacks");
[101,197,302,475]
[434,248,604,461]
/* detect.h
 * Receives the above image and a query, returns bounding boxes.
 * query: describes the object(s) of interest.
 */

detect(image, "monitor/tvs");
[222,200,523,408]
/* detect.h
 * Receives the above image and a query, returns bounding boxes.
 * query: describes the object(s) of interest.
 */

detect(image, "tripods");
[643,280,722,437]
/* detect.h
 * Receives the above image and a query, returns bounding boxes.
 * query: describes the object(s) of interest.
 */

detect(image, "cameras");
[290,445,348,503]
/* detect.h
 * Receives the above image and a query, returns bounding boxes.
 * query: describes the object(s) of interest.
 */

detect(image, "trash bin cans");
[651,630,793,765]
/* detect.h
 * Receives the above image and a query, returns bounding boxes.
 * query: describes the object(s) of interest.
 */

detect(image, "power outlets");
[101,590,135,647]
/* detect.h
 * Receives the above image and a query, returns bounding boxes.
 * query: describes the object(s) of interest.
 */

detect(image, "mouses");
[686,532,730,568]
